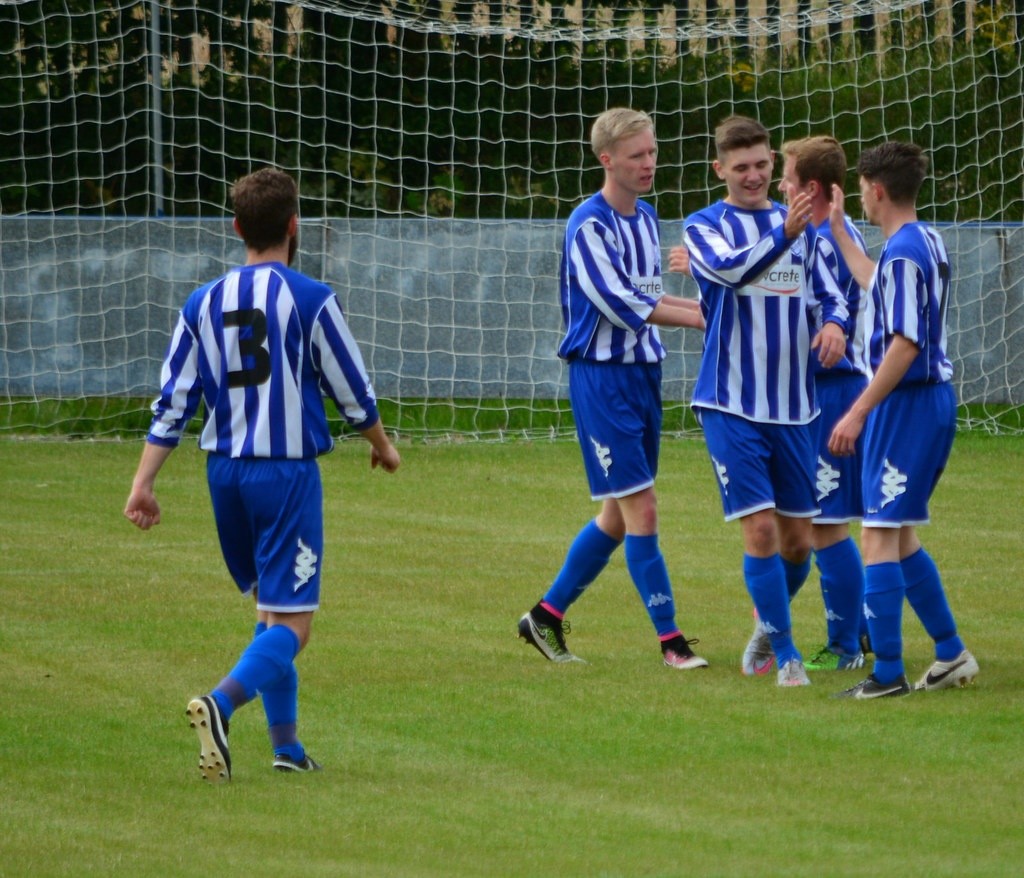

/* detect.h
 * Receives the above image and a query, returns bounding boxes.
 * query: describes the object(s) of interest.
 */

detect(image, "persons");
[682,116,851,686]
[667,134,871,670]
[124,169,402,783]
[829,142,980,699]
[516,108,709,669]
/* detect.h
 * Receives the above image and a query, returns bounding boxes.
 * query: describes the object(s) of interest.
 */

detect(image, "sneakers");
[185,697,232,786]
[776,658,812,687]
[858,634,872,656]
[660,634,710,671]
[741,626,777,682]
[829,679,913,703]
[913,650,982,693]
[801,647,866,674]
[518,606,588,664]
[273,748,323,776]
